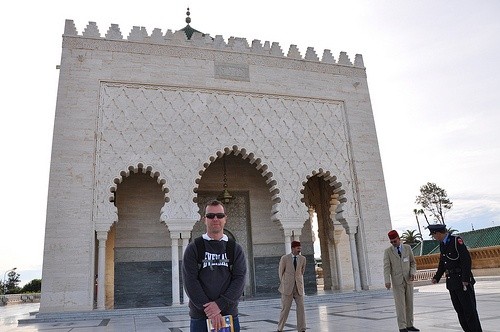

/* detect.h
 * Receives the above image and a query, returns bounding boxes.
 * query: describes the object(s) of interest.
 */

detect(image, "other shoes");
[407,326,419,331]
[400,329,408,332]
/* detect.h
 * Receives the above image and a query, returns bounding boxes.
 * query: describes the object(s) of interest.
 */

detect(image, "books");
[207,315,234,332]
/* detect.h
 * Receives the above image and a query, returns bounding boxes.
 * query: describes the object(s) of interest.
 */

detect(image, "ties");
[397,246,401,258]
[442,241,444,247]
[294,256,296,270]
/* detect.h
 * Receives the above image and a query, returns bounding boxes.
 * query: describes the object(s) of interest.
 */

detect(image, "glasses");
[206,213,225,219]
[390,238,398,244]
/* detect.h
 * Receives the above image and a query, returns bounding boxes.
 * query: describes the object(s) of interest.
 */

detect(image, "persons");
[427,225,483,332]
[182,200,247,332]
[384,230,420,332]
[278,240,306,332]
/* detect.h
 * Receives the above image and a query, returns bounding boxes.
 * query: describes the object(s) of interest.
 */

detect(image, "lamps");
[216,152,235,204]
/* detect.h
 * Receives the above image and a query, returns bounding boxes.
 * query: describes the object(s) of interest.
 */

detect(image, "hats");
[291,240,301,247]
[388,230,398,240]
[428,225,446,236]
